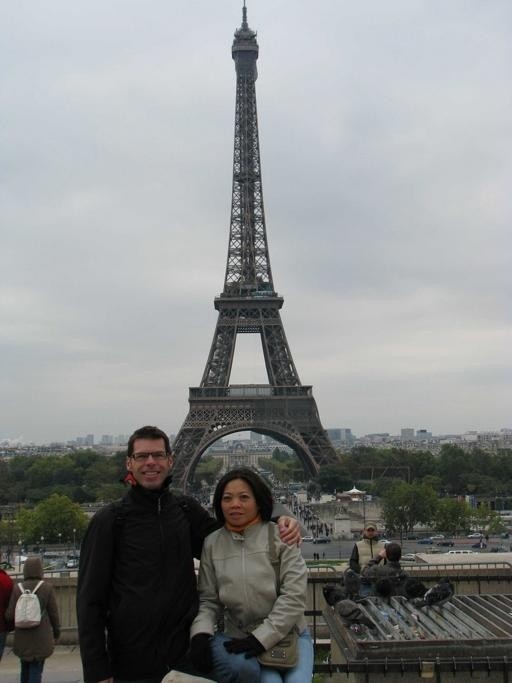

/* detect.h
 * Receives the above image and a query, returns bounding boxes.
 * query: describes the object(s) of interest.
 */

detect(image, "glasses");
[131,451,168,460]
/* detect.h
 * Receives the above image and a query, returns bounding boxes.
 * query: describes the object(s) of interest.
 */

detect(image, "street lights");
[359,493,370,531]
[58,534,61,560]
[73,528,77,551]
[18,541,22,573]
[41,536,44,564]
[395,505,411,549]
[286,491,320,539]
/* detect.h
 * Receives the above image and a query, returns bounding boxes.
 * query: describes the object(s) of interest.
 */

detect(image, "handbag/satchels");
[255,620,298,670]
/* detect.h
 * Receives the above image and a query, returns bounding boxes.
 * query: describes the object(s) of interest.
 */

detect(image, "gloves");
[190,633,212,674]
[223,635,265,659]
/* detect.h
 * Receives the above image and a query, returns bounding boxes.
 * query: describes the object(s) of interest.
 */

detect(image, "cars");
[376,531,512,561]
[14,546,80,570]
[313,536,331,544]
[301,536,314,542]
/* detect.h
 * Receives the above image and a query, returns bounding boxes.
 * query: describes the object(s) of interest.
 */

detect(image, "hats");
[364,523,377,531]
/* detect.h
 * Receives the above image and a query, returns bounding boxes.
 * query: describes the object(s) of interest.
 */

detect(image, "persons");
[349,522,389,598]
[485,534,489,542]
[76,425,303,683]
[10,548,60,683]
[193,468,314,682]
[362,544,408,600]
[275,498,333,537]
[0,551,14,666]
[313,551,325,560]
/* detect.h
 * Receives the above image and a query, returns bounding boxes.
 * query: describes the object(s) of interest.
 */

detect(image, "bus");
[288,481,304,488]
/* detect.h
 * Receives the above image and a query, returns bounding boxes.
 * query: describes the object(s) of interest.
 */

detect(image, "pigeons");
[372,575,394,596]
[415,578,454,609]
[320,584,369,606]
[399,570,429,604]
[334,599,378,627]
[343,568,361,596]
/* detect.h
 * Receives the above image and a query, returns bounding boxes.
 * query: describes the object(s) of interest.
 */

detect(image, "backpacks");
[14,580,44,628]
